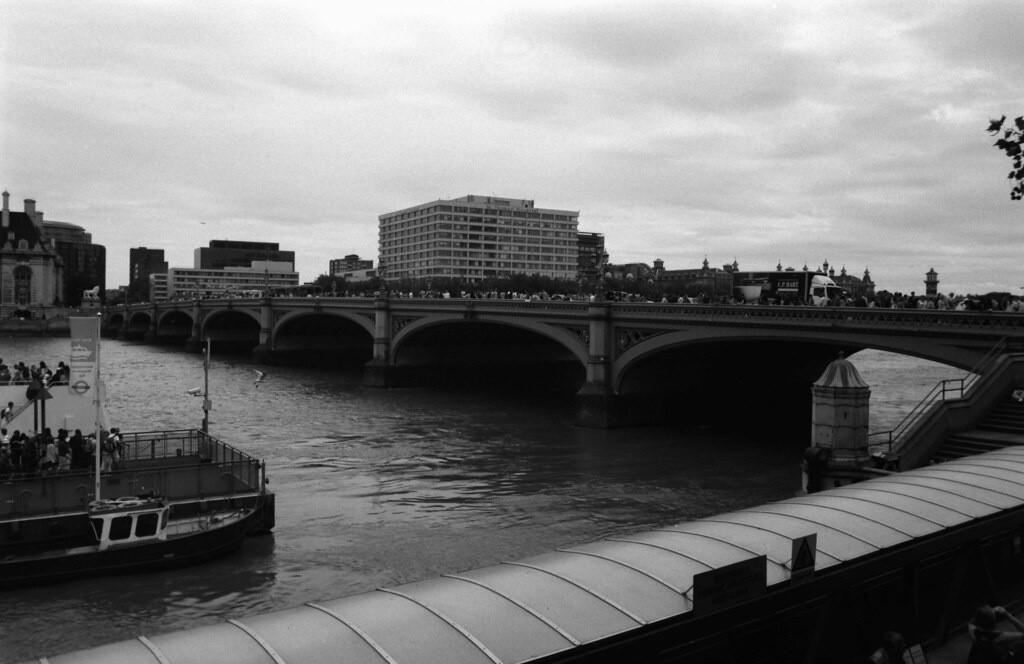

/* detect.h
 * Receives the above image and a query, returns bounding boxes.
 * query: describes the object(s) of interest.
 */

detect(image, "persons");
[0,357,130,476]
[967,605,1024,664]
[104,288,1024,311]
[868,631,909,664]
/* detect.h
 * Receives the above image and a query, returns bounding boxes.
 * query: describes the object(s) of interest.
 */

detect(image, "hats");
[59,362,64,367]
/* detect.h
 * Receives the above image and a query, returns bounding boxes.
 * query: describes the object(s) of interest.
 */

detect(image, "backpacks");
[85,438,94,452]
[105,435,116,451]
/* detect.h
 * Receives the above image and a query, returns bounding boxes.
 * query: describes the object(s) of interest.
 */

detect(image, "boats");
[0,311,276,586]
